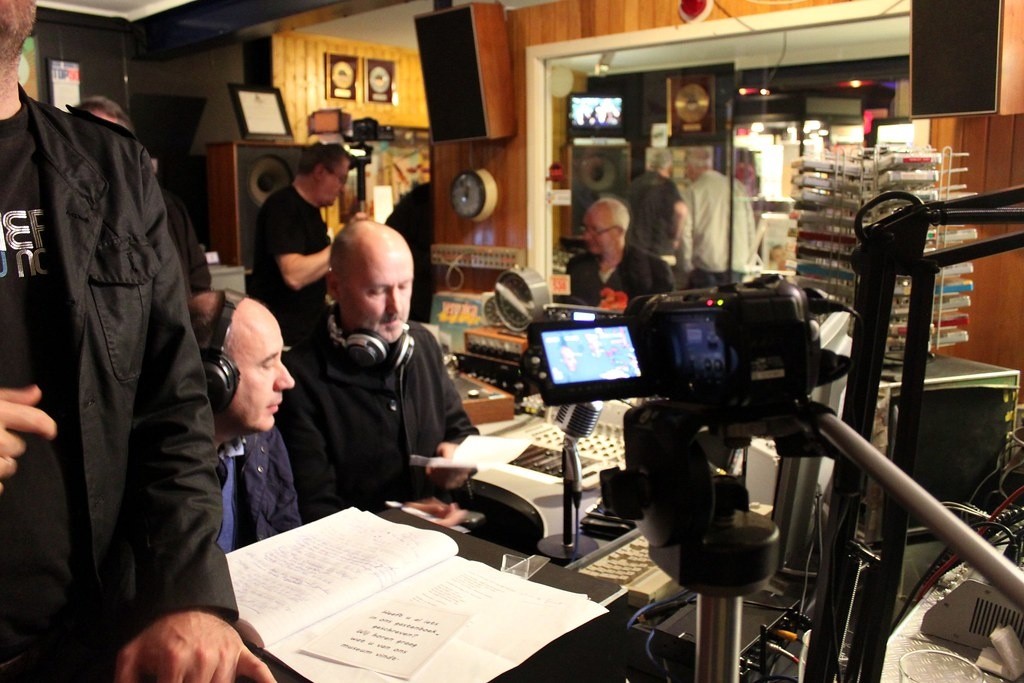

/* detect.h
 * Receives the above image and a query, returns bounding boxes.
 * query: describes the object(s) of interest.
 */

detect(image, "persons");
[187,288,301,554]
[253,142,354,358]
[0,0,277,683]
[272,220,481,527]
[683,145,754,286]
[624,146,688,289]
[67,97,211,301]
[566,198,676,309]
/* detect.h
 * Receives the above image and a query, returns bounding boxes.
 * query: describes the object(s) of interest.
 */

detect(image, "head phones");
[327,305,415,371]
[198,288,246,415]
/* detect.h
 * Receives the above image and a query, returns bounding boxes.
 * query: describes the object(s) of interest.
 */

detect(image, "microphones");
[549,400,603,508]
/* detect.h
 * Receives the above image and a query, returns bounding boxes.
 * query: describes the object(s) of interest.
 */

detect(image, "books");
[224,506,609,683]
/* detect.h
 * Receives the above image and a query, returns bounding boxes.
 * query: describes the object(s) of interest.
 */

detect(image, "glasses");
[579,223,623,236]
[325,165,348,185]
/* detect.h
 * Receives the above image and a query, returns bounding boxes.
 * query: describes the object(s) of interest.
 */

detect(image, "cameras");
[527,275,823,408]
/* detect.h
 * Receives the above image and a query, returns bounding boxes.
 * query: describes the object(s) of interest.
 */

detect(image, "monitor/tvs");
[567,93,626,138]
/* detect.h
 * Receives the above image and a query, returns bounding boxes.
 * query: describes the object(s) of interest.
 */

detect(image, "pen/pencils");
[385,501,472,534]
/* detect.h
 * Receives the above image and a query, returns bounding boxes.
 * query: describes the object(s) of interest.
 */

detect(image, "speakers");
[414,3,515,145]
[570,141,631,238]
[909,1,1023,120]
[205,140,306,273]
